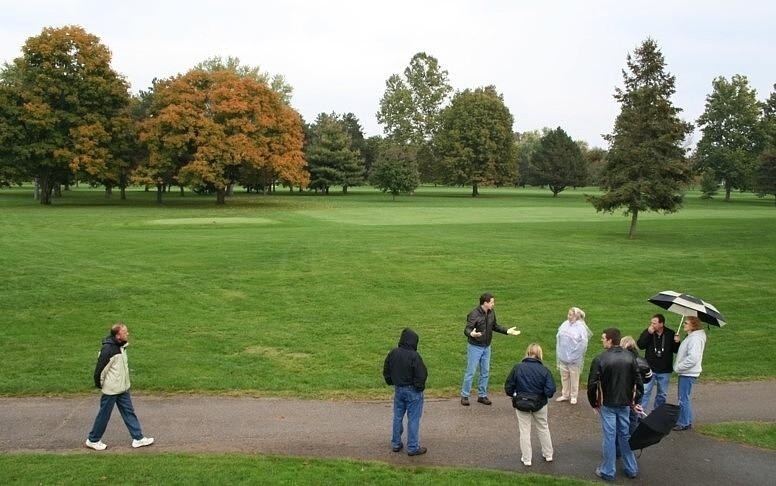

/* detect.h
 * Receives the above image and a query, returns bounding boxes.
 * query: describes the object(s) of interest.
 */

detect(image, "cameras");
[654,347,664,359]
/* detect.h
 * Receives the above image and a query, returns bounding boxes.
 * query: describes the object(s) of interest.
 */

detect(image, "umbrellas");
[645,289,728,336]
[627,400,683,459]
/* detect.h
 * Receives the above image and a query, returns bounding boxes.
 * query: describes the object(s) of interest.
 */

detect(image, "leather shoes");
[672,425,689,432]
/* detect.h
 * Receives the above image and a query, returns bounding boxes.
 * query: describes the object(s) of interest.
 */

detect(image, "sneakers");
[521,458,531,466]
[85,438,108,450]
[407,446,427,456]
[477,396,492,405]
[460,397,470,406]
[393,441,404,452]
[555,395,570,402]
[131,436,155,449]
[594,465,603,479]
[543,454,552,461]
[570,396,578,405]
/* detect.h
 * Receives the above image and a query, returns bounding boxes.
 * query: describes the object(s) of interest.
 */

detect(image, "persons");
[672,314,709,435]
[620,335,655,439]
[584,325,645,480]
[84,319,155,453]
[640,313,681,418]
[503,339,560,468]
[379,325,429,458]
[553,306,595,404]
[457,288,525,410]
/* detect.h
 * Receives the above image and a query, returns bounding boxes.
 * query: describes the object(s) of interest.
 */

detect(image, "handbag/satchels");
[512,390,542,412]
